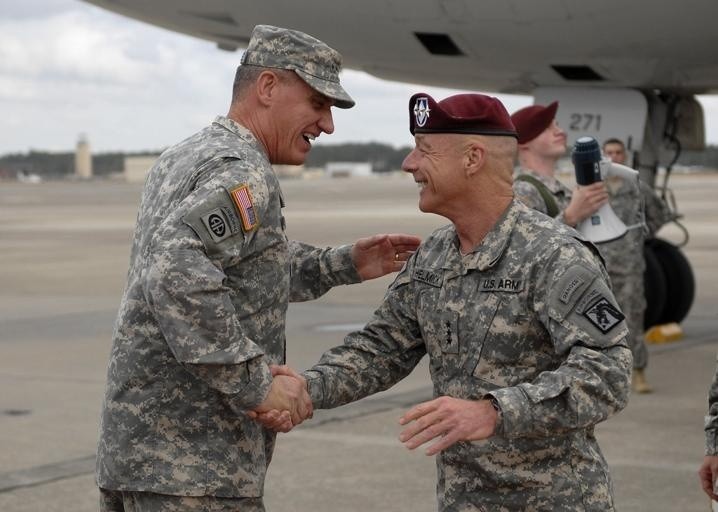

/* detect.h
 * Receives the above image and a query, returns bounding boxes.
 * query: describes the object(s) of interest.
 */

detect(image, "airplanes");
[79,0,718,331]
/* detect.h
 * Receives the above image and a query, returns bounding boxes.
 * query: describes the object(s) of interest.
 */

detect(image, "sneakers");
[632,367,652,396]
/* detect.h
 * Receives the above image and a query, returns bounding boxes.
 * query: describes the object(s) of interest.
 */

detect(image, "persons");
[395,253,399,260]
[697,357,718,512]
[250,89,632,511]
[92,25,422,511]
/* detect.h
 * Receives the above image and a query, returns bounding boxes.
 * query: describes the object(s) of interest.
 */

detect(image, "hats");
[512,99,560,145]
[409,89,520,139]
[241,25,355,111]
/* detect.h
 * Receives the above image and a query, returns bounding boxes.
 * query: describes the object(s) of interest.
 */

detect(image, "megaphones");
[571,136,639,245]
